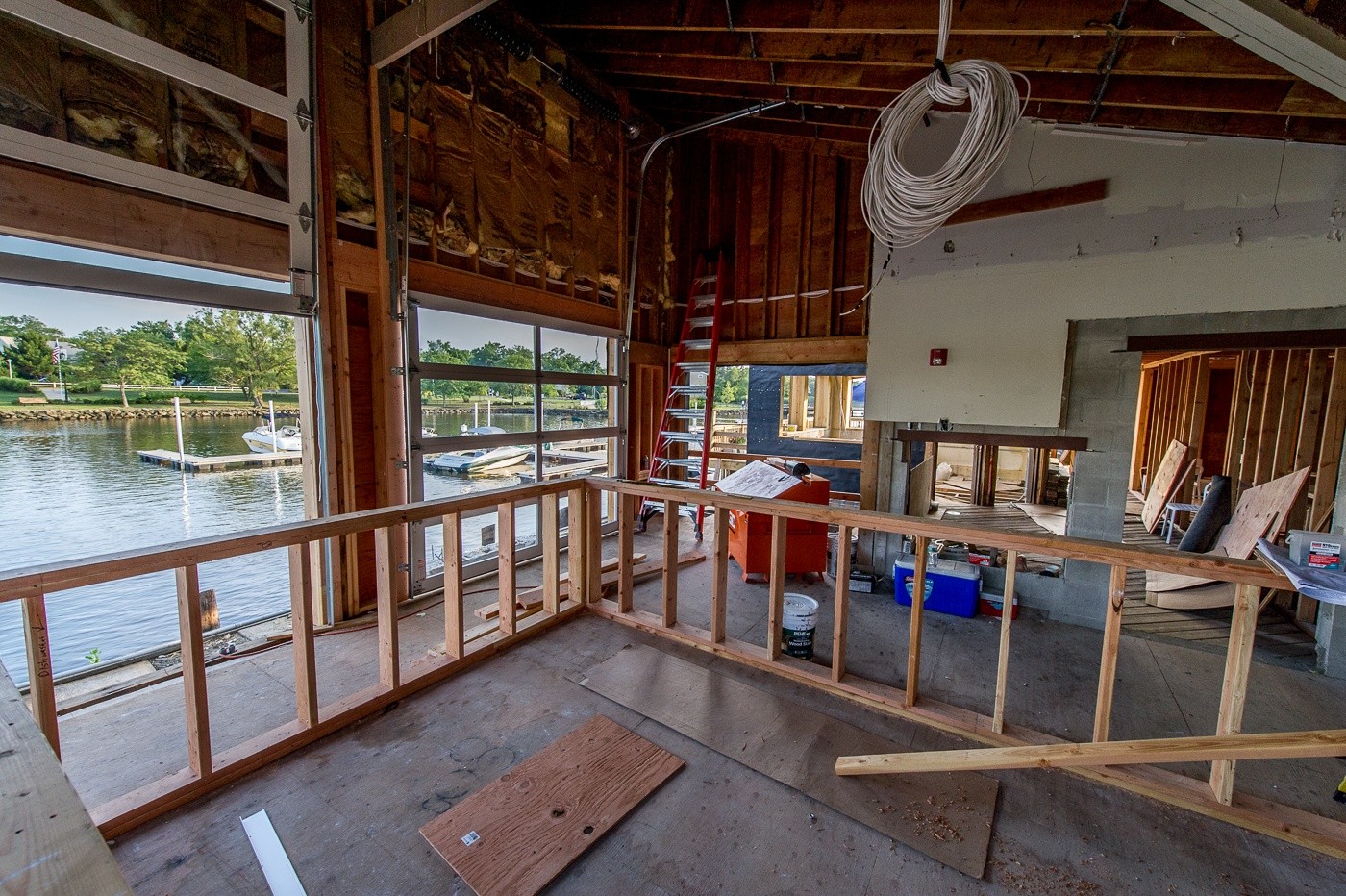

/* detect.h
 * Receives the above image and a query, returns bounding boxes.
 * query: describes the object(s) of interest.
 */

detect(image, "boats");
[423,400,532,473]
[555,414,584,424]
[241,418,439,453]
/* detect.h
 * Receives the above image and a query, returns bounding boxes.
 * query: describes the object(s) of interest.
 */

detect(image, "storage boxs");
[979,588,1020,620]
[892,554,984,619]
[1285,530,1345,570]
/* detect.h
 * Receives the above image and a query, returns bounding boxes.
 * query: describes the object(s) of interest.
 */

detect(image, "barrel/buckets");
[827,532,858,578]
[781,593,819,662]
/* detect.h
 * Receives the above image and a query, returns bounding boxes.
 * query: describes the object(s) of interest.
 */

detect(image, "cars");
[569,394,587,400]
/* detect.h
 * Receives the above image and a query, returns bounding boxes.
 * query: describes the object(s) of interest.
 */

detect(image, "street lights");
[594,366,597,401]
[6,358,12,379]
[512,384,515,406]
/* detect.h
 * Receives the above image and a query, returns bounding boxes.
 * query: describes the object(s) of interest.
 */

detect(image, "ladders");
[634,248,723,540]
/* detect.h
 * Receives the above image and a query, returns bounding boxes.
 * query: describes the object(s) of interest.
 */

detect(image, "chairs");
[1160,483,1213,544]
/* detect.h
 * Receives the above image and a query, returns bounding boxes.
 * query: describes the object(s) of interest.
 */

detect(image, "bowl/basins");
[1045,565,1058,573]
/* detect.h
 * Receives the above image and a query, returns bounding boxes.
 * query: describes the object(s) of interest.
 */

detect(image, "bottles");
[927,539,938,568]
